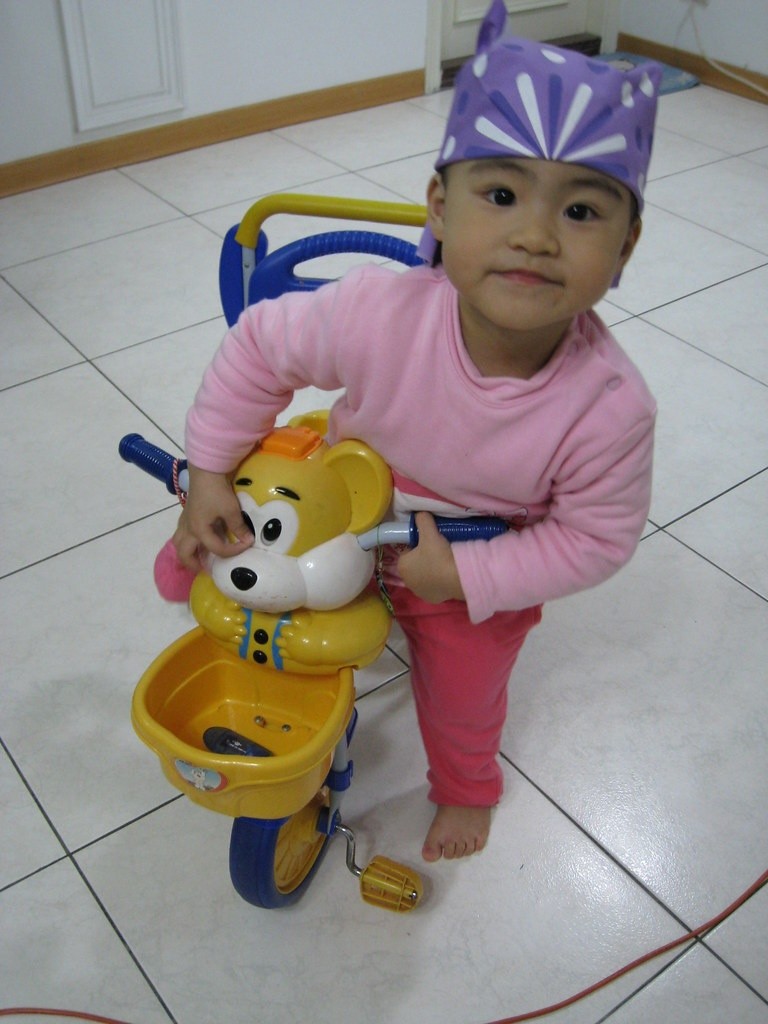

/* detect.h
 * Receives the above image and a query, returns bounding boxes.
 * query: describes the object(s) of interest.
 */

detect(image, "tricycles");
[117,192,512,911]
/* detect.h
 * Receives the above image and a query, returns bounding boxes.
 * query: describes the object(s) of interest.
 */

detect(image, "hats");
[435,0,663,216]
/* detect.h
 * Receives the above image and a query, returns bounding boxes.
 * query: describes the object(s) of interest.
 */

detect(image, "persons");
[155,37,662,863]
